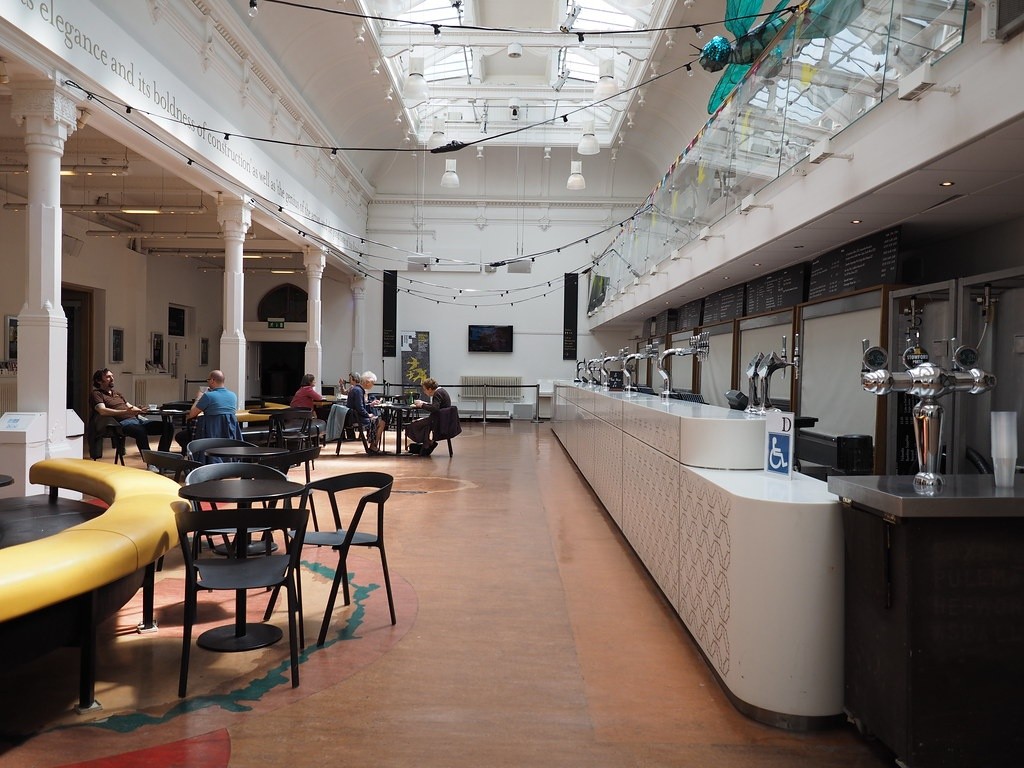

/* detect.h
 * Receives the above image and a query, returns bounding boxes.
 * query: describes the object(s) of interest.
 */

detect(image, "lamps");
[578,106,600,154]
[621,287,634,295]
[594,307,603,314]
[898,63,959,103]
[670,250,692,262]
[700,227,724,243]
[402,0,427,97]
[479,102,490,135]
[567,144,585,190]
[634,278,649,287]
[810,139,852,166]
[610,295,622,303]
[560,1,580,34]
[441,151,458,189]
[649,264,668,277]
[593,34,618,102]
[428,50,448,153]
[588,312,595,319]
[741,194,773,213]
[552,48,569,91]
[602,303,613,308]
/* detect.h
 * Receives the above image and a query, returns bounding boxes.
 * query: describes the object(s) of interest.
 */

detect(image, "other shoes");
[426,443,438,456]
[421,441,438,456]
[369,448,386,455]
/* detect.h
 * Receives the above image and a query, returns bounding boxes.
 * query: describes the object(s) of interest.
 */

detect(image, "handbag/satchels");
[406,443,423,454]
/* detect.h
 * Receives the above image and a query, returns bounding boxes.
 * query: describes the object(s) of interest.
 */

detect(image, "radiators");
[459,377,522,399]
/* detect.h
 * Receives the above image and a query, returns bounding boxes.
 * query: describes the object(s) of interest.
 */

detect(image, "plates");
[147,409,160,411]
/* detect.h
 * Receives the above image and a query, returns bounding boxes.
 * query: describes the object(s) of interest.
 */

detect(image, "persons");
[405,378,451,456]
[289,374,323,419]
[90,367,177,475]
[339,372,361,396]
[346,371,386,456]
[175,369,238,452]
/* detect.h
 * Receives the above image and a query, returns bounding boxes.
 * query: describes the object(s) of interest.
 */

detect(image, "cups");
[991,411,1018,488]
[149,404,157,411]
[200,386,208,396]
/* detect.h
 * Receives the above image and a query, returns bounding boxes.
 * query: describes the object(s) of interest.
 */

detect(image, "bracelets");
[195,398,199,400]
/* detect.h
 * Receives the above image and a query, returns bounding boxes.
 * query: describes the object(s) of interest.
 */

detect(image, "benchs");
[197,401,290,432]
[1,458,180,760]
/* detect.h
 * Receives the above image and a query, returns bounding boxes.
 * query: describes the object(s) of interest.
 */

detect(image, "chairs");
[90,393,462,696]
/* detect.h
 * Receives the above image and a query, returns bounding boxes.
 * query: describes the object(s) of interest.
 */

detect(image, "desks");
[316,399,346,443]
[178,482,304,652]
[139,410,190,456]
[202,448,289,556]
[372,405,419,457]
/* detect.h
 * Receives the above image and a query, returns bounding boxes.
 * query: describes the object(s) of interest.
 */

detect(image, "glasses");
[207,379,215,382]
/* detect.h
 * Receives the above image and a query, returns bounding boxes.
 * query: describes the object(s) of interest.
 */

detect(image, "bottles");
[408,392,414,405]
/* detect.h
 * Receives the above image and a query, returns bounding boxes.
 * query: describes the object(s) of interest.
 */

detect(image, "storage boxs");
[509,403,533,420]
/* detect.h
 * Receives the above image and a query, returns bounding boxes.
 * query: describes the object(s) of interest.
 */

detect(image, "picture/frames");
[151,332,165,365]
[4,315,17,361]
[200,337,209,365]
[109,327,125,364]
